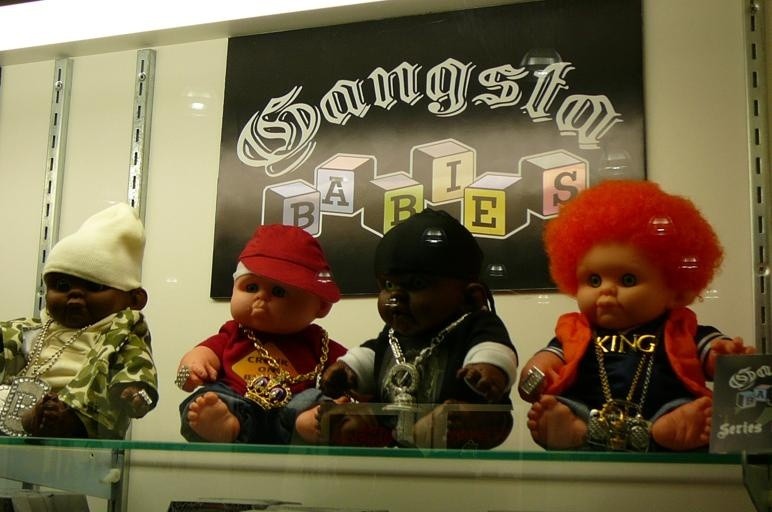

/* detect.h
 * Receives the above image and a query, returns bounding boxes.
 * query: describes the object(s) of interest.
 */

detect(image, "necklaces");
[0,316,94,436]
[237,320,329,412]
[387,312,473,446]
[586,325,663,451]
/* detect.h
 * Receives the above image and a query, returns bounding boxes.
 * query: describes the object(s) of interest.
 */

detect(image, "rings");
[175,364,193,391]
[130,388,153,407]
[520,365,546,394]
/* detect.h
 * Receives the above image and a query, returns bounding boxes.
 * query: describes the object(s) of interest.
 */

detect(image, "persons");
[0,202,161,439]
[317,207,522,450]
[517,180,763,455]
[171,222,348,445]
